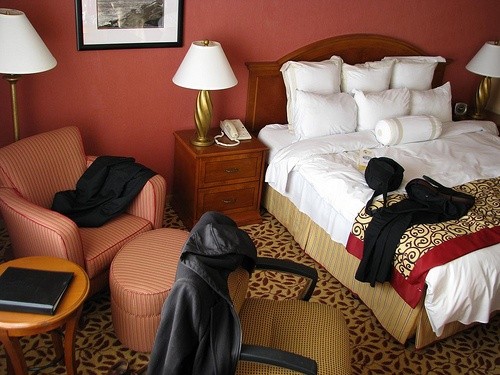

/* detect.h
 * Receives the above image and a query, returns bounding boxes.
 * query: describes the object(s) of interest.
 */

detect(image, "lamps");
[0,8,58,142]
[171,39,239,146]
[464,41,500,120]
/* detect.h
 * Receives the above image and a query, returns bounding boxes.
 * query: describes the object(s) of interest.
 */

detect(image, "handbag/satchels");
[365,157,405,216]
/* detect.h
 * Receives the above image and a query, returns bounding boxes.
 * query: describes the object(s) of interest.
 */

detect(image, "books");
[0,267,75,316]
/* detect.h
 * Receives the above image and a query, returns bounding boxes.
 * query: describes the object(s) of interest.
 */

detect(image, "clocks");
[453,102,468,116]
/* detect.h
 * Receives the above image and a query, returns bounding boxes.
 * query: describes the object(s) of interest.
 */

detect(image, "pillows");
[352,88,410,132]
[408,80,452,122]
[374,114,443,146]
[279,55,344,134]
[341,58,400,98]
[381,56,446,91]
[292,88,357,143]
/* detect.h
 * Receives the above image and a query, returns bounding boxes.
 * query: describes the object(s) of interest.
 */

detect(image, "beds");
[245,33,500,350]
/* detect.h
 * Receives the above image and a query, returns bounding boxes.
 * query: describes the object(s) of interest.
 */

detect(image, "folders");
[0,267,74,316]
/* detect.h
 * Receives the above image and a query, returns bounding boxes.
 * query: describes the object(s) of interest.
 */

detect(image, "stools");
[109,228,190,353]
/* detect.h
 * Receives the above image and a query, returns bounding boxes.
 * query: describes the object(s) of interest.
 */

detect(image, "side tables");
[0,255,92,375]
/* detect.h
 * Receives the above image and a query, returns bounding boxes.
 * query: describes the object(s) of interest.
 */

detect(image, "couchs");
[0,126,167,331]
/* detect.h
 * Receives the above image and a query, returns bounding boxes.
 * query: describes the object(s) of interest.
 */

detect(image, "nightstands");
[169,126,271,232]
[451,107,500,137]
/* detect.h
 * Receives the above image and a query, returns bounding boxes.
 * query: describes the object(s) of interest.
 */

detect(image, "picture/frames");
[75,0,185,52]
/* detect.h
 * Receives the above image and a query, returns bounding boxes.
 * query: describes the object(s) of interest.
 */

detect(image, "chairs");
[146,211,350,375]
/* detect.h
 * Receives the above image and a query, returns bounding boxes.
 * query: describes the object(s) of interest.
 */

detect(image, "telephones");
[220,119,252,141]
